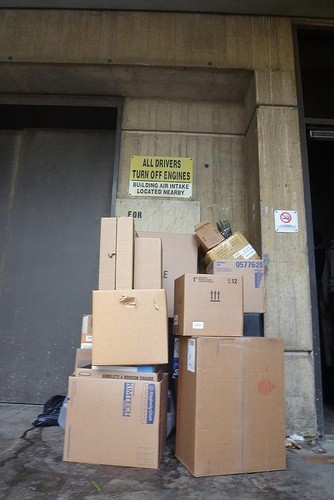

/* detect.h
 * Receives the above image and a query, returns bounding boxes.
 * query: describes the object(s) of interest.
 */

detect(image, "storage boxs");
[173,272,245,338]
[61,368,171,470]
[90,288,170,366]
[205,259,268,315]
[194,217,225,253]
[131,235,165,290]
[202,231,261,269]
[137,231,199,319]
[174,335,288,479]
[97,216,137,290]
